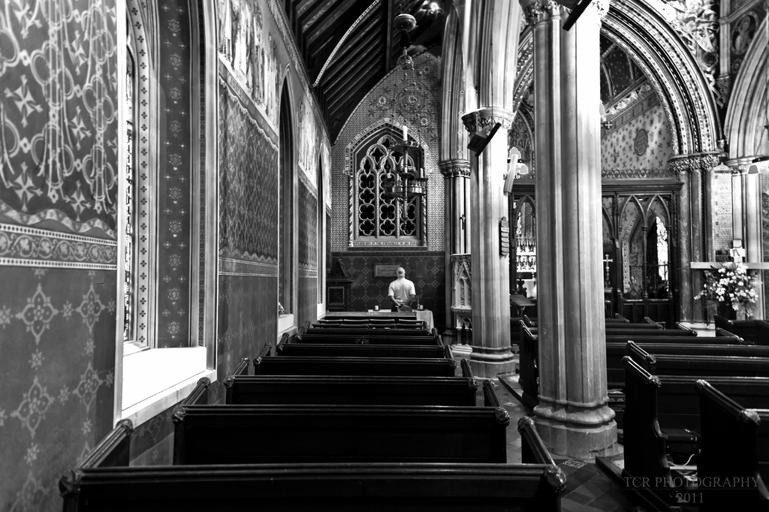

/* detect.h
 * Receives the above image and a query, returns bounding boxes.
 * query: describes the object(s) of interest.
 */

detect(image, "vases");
[718,301,737,321]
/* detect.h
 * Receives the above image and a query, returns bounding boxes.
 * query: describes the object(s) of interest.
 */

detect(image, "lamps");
[382,12,429,218]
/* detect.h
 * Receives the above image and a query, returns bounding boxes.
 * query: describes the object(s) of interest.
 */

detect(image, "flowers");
[693,261,764,317]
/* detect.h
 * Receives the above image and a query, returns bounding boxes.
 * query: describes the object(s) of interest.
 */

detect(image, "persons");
[531,280,537,298]
[387,266,416,312]
